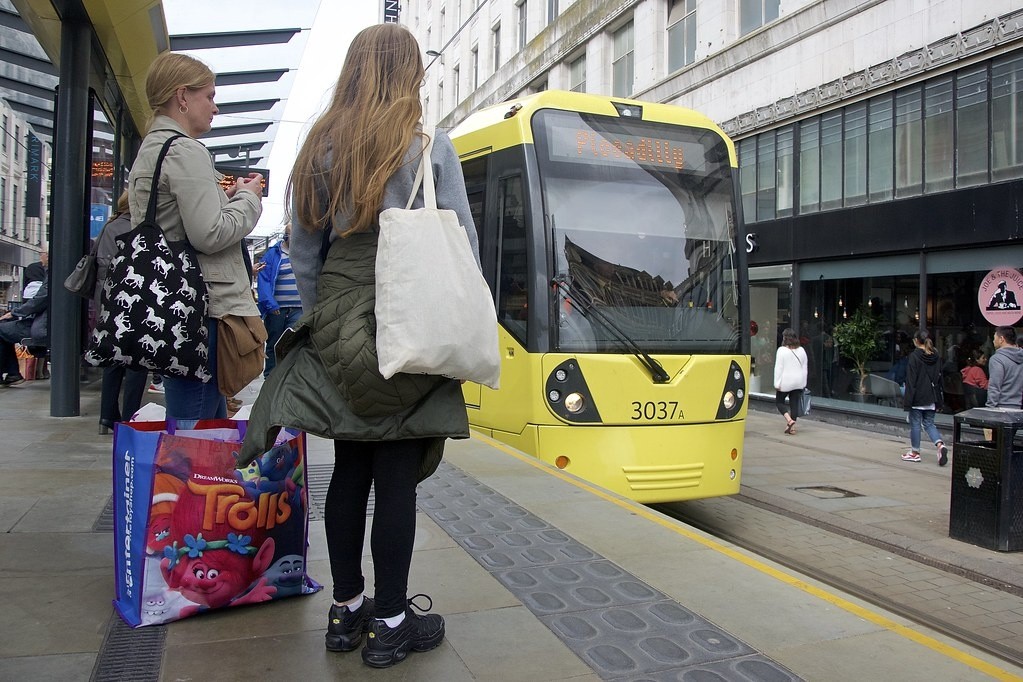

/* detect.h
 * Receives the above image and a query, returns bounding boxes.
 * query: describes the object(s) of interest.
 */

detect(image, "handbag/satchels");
[374,125,503,390]
[85,134,211,383]
[112,416,325,628]
[17,345,37,380]
[129,402,166,422]
[933,385,945,403]
[797,388,811,416]
[217,314,270,397]
[63,221,112,300]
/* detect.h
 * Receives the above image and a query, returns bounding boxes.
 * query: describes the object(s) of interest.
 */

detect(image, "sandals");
[785,420,795,433]
[789,430,795,435]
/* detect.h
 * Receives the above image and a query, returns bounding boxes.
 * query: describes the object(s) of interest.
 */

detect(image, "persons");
[901,330,948,466]
[774,328,807,436]
[236,24,483,669]
[0,241,49,388]
[258,223,304,379]
[578,256,679,309]
[95,190,147,436]
[984,326,1023,409]
[942,345,988,390]
[129,53,263,419]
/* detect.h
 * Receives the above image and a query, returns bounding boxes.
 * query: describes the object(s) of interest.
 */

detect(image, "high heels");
[99,419,114,434]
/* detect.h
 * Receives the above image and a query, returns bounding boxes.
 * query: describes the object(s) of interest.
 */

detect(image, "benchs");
[21,337,49,347]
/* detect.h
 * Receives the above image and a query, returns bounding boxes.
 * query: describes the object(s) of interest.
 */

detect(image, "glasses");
[38,251,46,255]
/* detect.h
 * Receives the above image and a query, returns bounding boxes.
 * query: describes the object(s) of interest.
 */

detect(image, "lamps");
[426,50,445,65]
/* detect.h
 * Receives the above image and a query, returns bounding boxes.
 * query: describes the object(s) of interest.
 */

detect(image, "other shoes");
[0,373,24,386]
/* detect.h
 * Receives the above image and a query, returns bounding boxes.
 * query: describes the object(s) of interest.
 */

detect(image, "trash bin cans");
[949,407,1023,554]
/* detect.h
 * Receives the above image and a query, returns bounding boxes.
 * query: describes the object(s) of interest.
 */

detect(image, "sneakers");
[937,444,948,466]
[325,595,375,652]
[147,381,166,393]
[901,451,922,461]
[361,594,445,668]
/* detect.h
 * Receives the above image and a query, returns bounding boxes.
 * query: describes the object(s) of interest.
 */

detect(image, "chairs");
[869,374,901,407]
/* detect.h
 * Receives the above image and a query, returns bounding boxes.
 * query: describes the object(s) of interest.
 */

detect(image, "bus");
[443,89,750,504]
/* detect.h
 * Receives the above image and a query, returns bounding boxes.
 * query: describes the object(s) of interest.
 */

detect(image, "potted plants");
[830,301,889,403]
